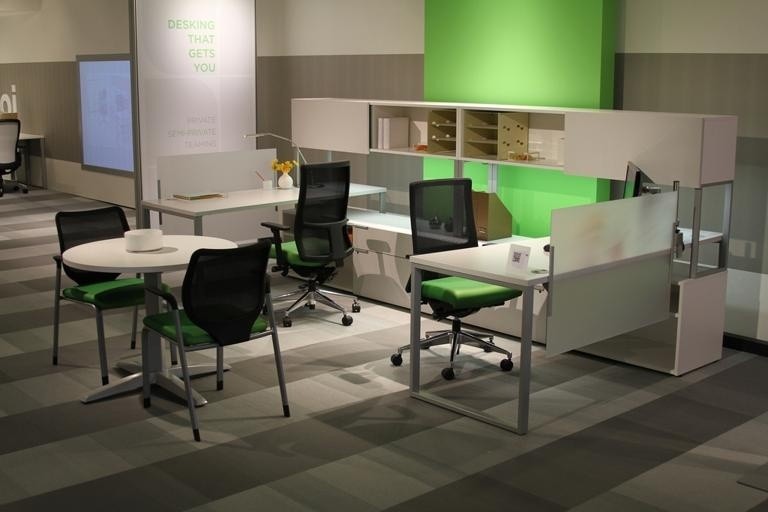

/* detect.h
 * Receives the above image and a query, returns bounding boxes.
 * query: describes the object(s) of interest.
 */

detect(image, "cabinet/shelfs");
[289,96,738,376]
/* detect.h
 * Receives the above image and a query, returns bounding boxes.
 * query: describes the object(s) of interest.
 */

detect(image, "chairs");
[0,117,30,195]
[252,160,363,328]
[137,232,292,441]
[49,206,176,387]
[392,178,522,379]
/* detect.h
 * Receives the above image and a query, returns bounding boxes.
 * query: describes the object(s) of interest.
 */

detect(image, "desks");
[142,181,387,233]
[10,133,48,189]
[65,233,240,406]
[409,226,723,434]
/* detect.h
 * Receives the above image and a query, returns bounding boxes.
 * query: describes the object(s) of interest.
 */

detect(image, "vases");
[278,174,294,190]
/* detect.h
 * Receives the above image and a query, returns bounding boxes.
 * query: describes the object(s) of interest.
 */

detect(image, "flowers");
[271,161,296,173]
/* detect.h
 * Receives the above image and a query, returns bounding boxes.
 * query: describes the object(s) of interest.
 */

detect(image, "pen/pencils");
[256,171,265,181]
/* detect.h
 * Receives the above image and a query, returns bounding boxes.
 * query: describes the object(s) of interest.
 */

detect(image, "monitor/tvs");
[622,160,642,198]
